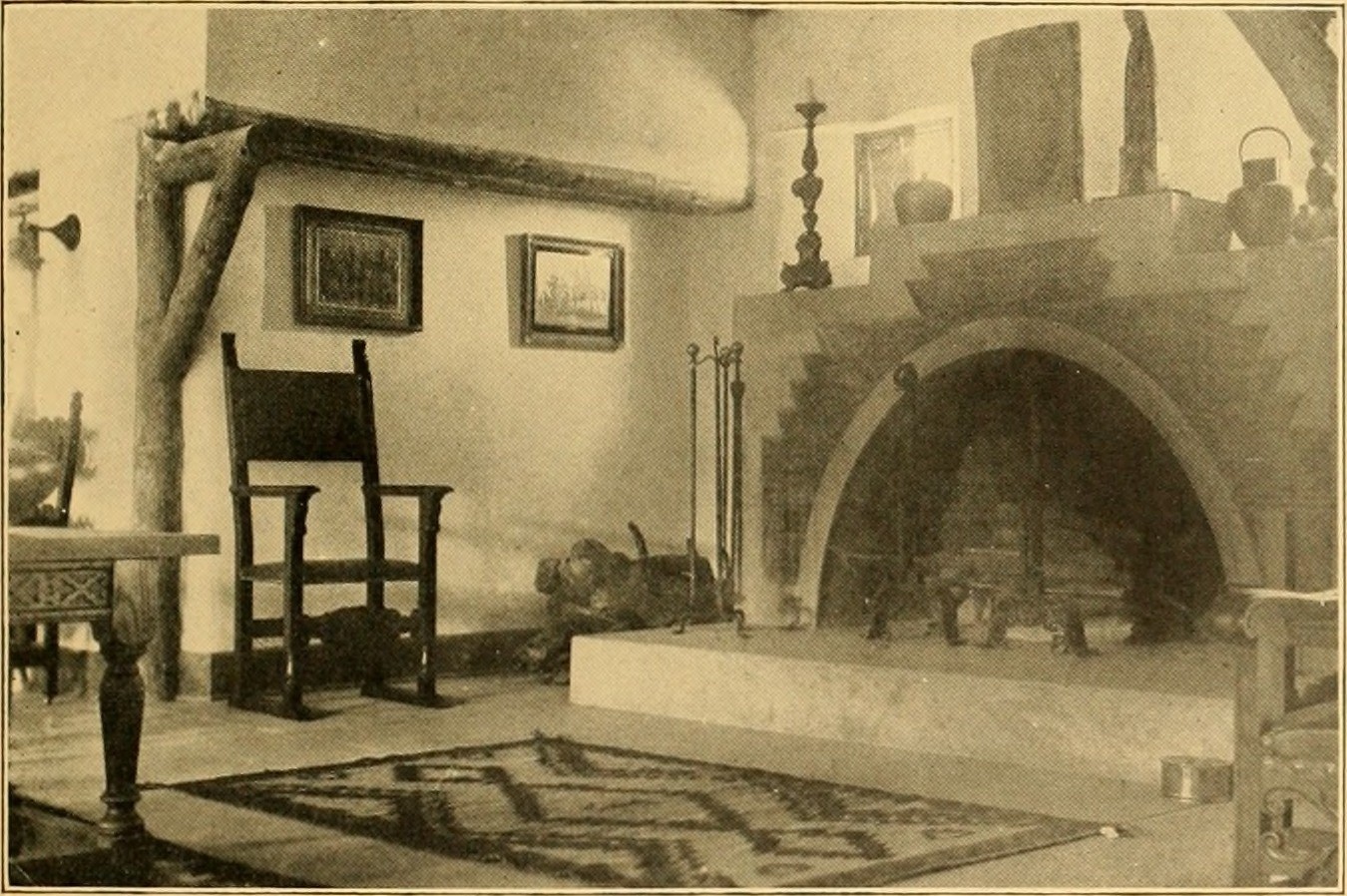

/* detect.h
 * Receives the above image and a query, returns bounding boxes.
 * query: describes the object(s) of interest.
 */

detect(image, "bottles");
[1228,128,1293,250]
[894,182,952,224]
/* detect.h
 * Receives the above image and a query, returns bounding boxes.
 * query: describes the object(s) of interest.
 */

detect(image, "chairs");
[220,332,454,721]
[1212,592,1339,888]
[9,391,82,699]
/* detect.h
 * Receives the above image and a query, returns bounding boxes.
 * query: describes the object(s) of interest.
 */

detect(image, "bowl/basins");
[1160,755,1235,804]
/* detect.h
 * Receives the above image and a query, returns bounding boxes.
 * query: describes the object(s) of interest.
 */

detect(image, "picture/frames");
[292,203,425,332]
[521,233,625,349]
[854,124,914,257]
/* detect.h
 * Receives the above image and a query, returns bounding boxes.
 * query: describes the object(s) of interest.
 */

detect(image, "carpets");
[9,790,334,888]
[170,729,1121,888]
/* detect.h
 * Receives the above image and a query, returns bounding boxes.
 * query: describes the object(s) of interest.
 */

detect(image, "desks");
[9,528,219,887]
